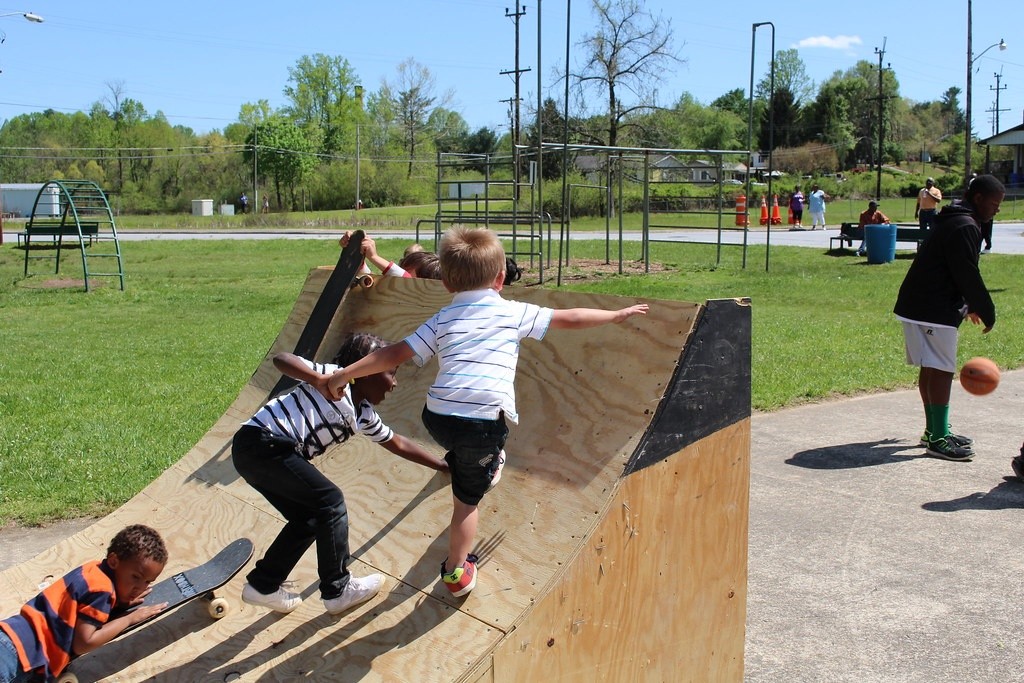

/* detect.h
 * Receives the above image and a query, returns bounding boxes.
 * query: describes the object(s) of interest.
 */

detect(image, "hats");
[869,201,880,208]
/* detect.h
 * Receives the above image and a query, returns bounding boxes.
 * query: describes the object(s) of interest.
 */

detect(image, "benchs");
[18,222,99,248]
[830,223,929,255]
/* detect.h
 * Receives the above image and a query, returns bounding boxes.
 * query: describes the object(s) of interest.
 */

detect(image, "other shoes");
[856,251,860,257]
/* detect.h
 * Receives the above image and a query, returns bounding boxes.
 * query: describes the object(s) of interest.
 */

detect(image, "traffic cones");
[759,195,769,225]
[771,194,783,225]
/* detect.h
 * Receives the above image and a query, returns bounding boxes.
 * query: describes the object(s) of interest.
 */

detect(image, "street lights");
[965,37,1008,179]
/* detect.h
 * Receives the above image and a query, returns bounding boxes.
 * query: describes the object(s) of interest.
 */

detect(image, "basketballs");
[959,356,1001,397]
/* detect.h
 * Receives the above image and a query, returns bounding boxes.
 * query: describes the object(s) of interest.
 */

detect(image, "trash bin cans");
[864,224,896,263]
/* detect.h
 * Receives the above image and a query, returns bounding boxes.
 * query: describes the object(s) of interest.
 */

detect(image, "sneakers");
[242,583,302,613]
[926,433,975,460]
[921,428,973,448]
[441,553,479,598]
[323,574,385,615]
[485,450,506,494]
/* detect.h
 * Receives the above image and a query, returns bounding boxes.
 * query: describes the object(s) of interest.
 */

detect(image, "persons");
[340,230,441,280]
[915,177,942,252]
[404,243,424,258]
[807,185,830,231]
[855,201,890,257]
[240,192,248,214]
[503,256,521,285]
[789,184,806,231]
[232,333,448,615]
[327,225,649,596]
[0,524,169,683]
[892,175,1006,463]
[262,193,268,214]
[359,199,362,209]
[1011,440,1024,486]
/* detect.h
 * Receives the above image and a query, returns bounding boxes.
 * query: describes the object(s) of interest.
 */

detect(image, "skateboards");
[286,227,375,363]
[43,539,254,682]
